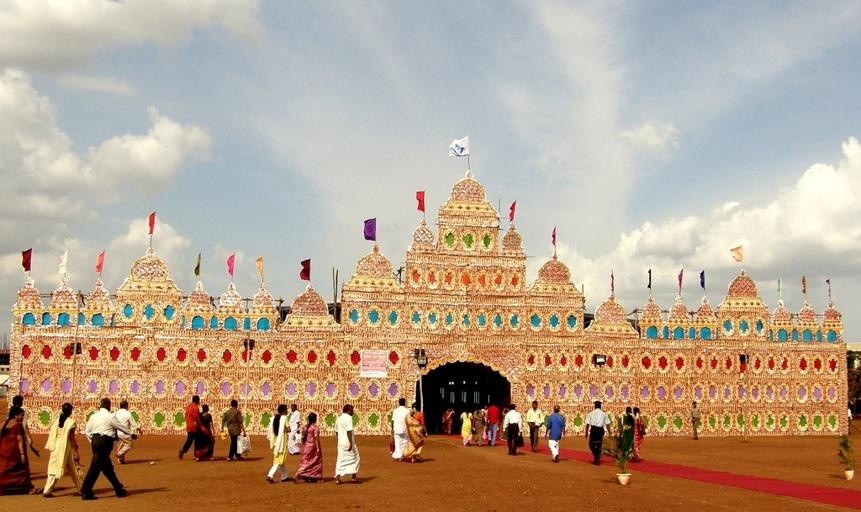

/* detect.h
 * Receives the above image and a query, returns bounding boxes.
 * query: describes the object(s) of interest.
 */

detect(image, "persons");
[0,408,44,494]
[43,401,81,497]
[391,399,646,465]
[11,397,33,446]
[193,254,263,276]
[848,406,853,434]
[116,399,142,464]
[686,402,701,439]
[148,212,155,234]
[81,399,138,499]
[21,248,104,277]
[178,396,362,485]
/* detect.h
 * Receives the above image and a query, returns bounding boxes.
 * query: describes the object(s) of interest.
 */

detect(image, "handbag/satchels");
[517,436,524,446]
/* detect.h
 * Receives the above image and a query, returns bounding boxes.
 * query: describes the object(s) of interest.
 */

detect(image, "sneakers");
[352,478,363,483]
[117,489,129,497]
[291,476,299,483]
[82,493,97,500]
[281,477,291,481]
[266,477,275,483]
[179,449,183,459]
[115,453,127,464]
[43,492,54,497]
[336,480,343,484]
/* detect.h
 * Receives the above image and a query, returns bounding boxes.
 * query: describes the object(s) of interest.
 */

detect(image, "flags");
[416,192,424,212]
[364,218,376,241]
[509,202,515,221]
[300,259,310,281]
[611,270,705,292]
[449,136,469,157]
[733,246,742,263]
[551,227,556,246]
[777,276,831,298]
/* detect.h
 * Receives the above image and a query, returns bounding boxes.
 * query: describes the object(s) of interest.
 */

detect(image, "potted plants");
[615,413,635,485]
[836,422,856,481]
